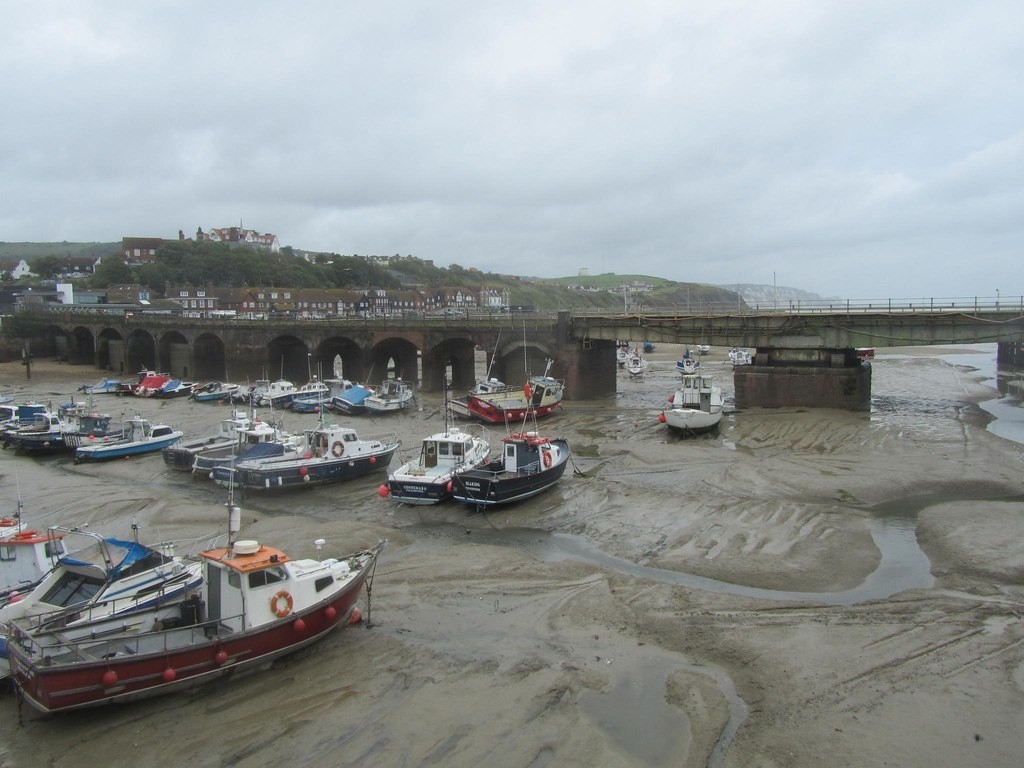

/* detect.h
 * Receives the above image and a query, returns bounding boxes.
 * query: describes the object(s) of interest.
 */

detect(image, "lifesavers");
[271,591,293,617]
[543,452,552,468]
[332,441,345,457]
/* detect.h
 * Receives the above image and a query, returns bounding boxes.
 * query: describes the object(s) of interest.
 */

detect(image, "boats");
[76,362,413,416]
[0,401,48,442]
[642,342,655,353]
[465,321,565,425]
[675,348,700,375]
[212,361,401,494]
[3,390,113,456]
[447,327,505,420]
[446,385,586,514]
[73,407,184,466]
[695,345,710,356]
[728,346,752,370]
[616,340,632,369]
[0,514,68,603]
[159,370,270,472]
[658,351,724,437]
[624,346,648,378]
[5,406,390,728]
[855,347,876,359]
[0,515,232,694]
[377,392,492,507]
[193,370,306,481]
